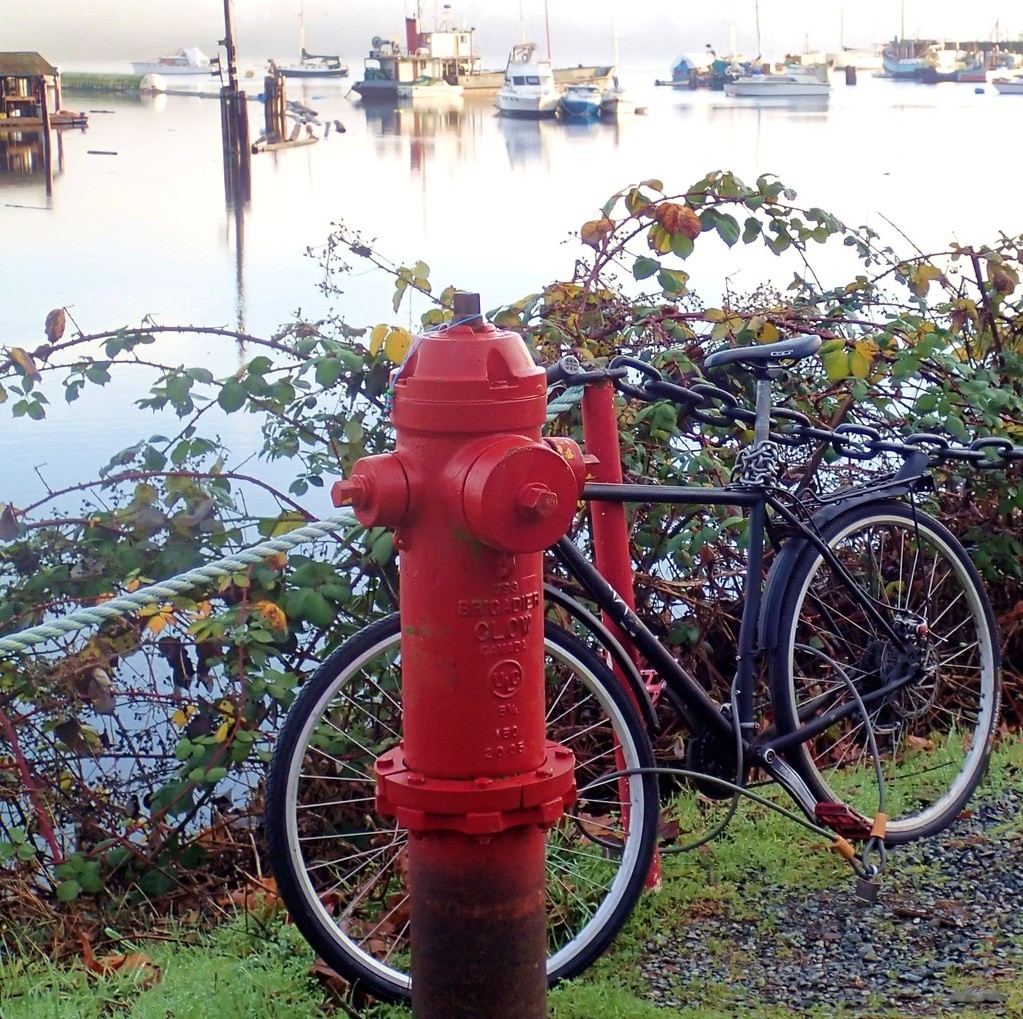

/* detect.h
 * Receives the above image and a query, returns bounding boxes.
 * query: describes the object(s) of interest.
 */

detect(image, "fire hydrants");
[329,288,602,1019]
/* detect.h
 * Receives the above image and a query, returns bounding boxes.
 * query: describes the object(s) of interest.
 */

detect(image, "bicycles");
[259,332,1006,1010]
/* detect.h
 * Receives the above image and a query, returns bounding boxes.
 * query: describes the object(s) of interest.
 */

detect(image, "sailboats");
[264,0,352,78]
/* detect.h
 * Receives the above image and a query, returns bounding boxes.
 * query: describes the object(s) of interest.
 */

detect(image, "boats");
[138,72,168,94]
[49,109,89,126]
[654,1,1023,95]
[351,1,622,125]
[130,45,219,77]
